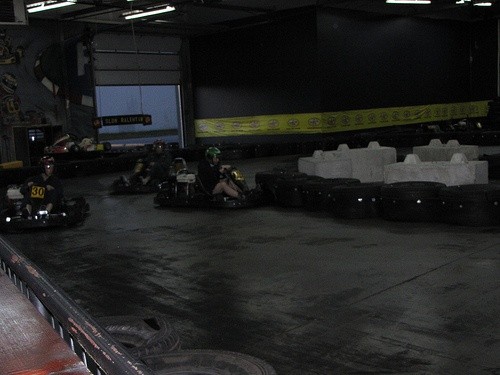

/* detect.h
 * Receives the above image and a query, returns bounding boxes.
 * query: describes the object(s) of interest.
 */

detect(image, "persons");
[198,147,254,202]
[20,138,187,216]
